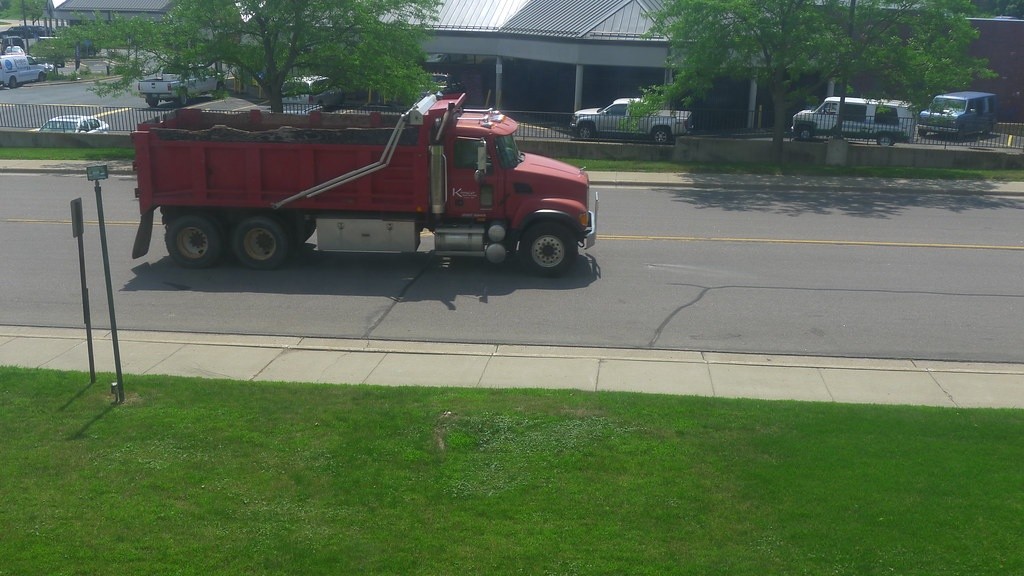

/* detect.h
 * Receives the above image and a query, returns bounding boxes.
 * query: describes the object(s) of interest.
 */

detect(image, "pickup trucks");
[568,96,695,147]
[136,61,226,108]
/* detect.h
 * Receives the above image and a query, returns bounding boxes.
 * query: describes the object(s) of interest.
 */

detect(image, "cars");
[282,73,342,111]
[28,113,110,137]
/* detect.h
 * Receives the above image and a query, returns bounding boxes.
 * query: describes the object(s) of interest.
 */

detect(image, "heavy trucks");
[130,89,599,278]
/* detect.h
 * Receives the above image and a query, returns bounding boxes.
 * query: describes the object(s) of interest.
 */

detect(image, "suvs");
[0,25,56,53]
[788,96,920,149]
[920,91,998,141]
[0,54,50,89]
